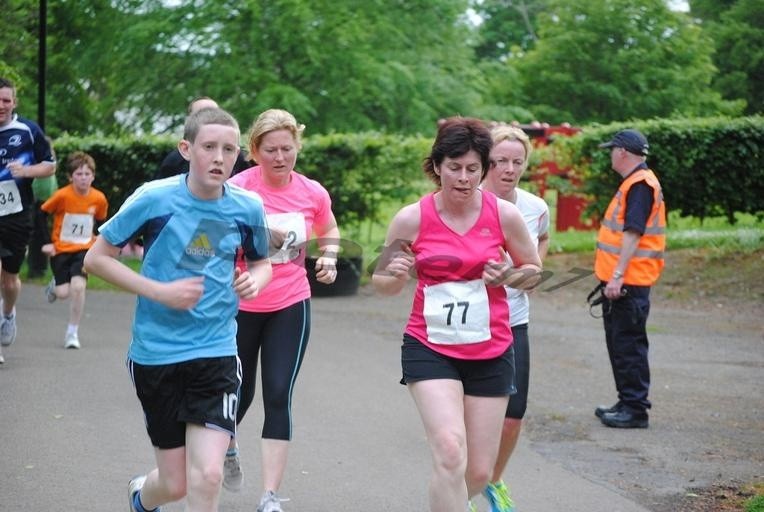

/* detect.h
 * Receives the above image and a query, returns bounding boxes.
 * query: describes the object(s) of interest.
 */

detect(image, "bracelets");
[612,270,623,280]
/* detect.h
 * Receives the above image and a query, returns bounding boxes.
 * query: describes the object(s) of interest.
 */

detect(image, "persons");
[373,118,543,511]
[23,173,60,283]
[477,127,552,511]
[0,77,58,366]
[590,128,667,429]
[224,109,343,512]
[81,105,275,512]
[154,96,253,181]
[34,151,110,351]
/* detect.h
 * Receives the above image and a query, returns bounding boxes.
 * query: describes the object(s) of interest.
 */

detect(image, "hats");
[597,129,648,156]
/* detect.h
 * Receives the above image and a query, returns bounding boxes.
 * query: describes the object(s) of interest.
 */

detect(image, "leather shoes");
[603,407,648,429]
[595,402,623,418]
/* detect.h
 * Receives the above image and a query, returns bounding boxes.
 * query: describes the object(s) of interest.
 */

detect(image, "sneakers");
[47,277,57,303]
[255,491,289,512]
[1,299,17,346]
[126,475,160,512]
[466,480,517,511]
[221,443,244,493]
[63,333,80,349]
